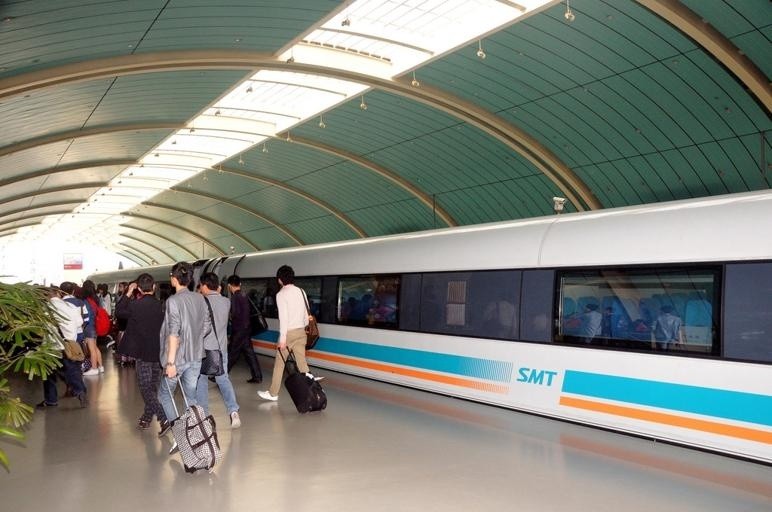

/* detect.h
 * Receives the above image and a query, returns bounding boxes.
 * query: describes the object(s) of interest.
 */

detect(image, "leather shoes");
[170,442,178,455]
[246,378,263,384]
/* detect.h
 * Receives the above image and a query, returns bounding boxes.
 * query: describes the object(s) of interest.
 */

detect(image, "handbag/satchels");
[63,339,85,361]
[96,307,112,336]
[200,350,226,375]
[305,315,319,350]
[248,314,268,336]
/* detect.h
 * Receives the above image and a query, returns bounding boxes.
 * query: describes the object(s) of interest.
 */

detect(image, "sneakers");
[230,412,241,429]
[136,420,151,430]
[80,392,89,407]
[158,420,171,437]
[83,366,106,375]
[257,390,278,401]
[36,400,59,409]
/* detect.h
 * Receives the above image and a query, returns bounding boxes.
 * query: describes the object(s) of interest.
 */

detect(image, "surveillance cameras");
[229,245,235,253]
[552,196,566,212]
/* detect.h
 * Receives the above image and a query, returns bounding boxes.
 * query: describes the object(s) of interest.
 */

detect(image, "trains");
[84,190,772,464]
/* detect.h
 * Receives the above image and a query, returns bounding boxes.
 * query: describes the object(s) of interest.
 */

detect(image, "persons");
[111,273,172,437]
[564,303,619,338]
[651,304,684,350]
[264,287,274,318]
[340,284,398,325]
[254,262,313,403]
[627,318,652,341]
[156,260,213,455]
[32,270,263,410]
[198,271,243,431]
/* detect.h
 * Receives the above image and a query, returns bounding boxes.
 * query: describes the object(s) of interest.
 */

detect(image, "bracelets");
[166,360,177,367]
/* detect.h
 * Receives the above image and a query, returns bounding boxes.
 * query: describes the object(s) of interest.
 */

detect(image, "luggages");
[163,373,220,474]
[277,345,327,413]
